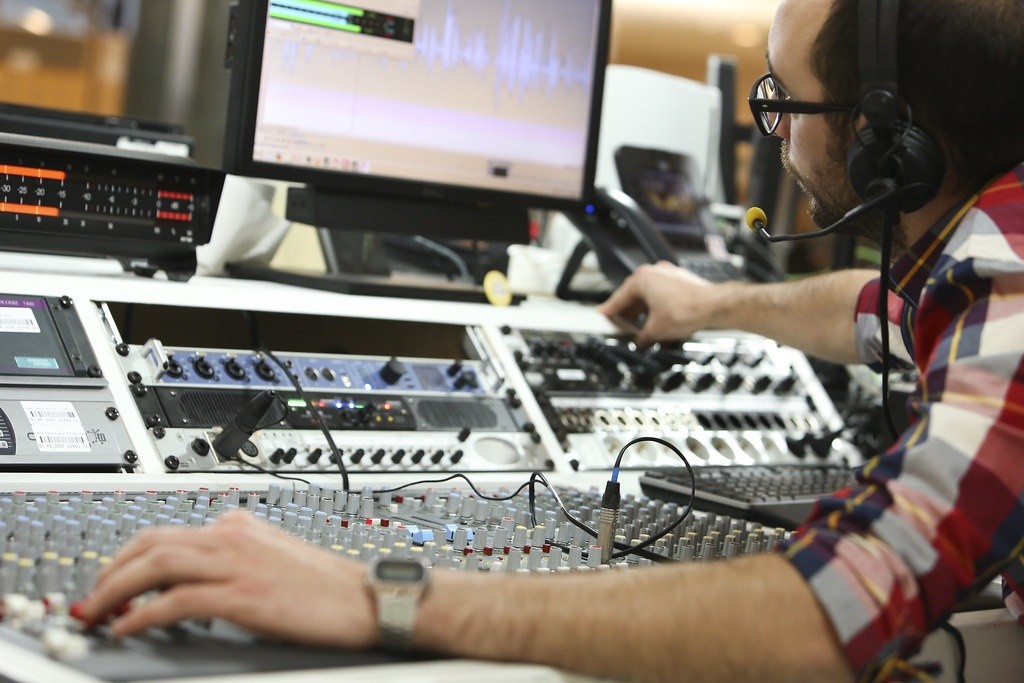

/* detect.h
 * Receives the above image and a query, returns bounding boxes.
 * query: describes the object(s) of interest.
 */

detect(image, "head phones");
[845,0,949,222]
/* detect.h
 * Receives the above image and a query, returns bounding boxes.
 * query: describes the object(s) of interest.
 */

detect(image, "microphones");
[742,203,872,241]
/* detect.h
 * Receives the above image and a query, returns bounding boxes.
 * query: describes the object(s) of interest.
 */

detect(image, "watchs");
[365,557,433,649]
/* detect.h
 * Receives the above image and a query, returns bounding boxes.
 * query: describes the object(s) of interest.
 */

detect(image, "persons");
[68,0,1024,683]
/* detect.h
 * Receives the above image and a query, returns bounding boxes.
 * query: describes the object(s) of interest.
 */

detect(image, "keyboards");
[638,465,863,533]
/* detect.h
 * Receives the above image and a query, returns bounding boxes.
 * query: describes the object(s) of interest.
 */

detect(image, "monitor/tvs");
[220,0,737,295]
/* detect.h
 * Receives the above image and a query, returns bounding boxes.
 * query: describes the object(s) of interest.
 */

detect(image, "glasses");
[748,73,864,137]
